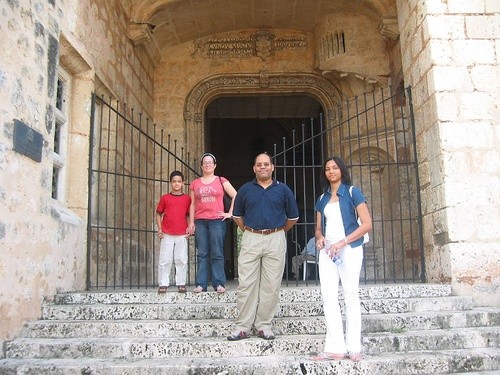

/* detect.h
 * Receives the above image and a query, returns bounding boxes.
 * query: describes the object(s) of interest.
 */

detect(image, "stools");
[303,261,320,280]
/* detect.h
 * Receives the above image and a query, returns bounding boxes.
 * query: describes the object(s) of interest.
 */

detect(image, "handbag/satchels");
[219,177,233,223]
[349,186,369,243]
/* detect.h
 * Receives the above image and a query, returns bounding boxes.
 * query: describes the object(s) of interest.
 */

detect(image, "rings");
[333,249,336,252]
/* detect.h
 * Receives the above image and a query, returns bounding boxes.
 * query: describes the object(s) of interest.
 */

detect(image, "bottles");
[324,243,342,265]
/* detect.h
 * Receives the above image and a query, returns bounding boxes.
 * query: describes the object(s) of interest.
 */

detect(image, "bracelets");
[343,238,347,246]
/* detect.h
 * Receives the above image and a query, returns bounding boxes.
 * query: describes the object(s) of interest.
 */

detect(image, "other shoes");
[259,329,275,339]
[216,285,225,292]
[194,286,203,292]
[227,330,250,341]
[178,285,188,292]
[159,286,167,292]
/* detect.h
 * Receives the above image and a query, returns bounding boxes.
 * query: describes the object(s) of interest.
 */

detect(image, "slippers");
[349,352,361,362]
[310,353,342,360]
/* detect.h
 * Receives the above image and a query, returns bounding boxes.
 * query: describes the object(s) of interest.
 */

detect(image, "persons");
[156,171,192,292]
[227,153,300,341]
[292,231,315,280]
[188,152,238,293]
[311,156,372,361]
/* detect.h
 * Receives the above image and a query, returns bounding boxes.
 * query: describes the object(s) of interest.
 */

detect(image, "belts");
[245,226,283,235]
